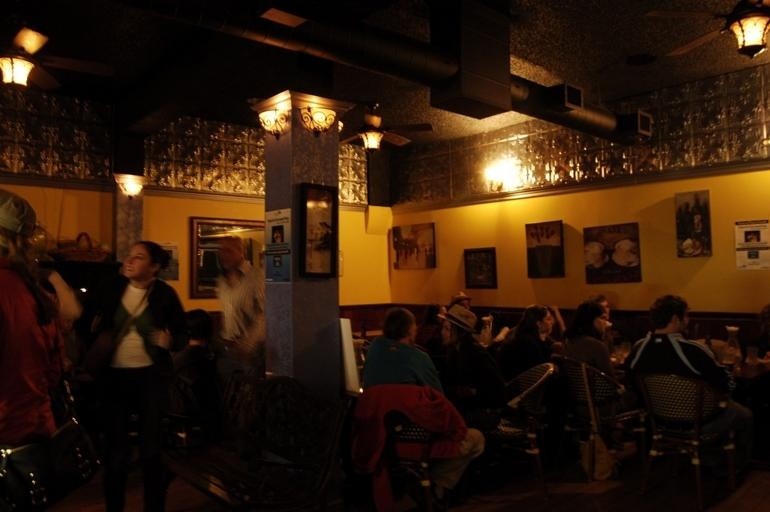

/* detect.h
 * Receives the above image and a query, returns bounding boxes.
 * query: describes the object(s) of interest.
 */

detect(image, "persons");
[32,224,266,512]
[358,294,732,460]
[0,187,85,511]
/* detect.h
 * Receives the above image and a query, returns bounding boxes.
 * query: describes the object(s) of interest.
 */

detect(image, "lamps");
[724,2,768,58]
[110,172,147,198]
[360,123,389,151]
[1,43,35,89]
[255,110,291,139]
[300,104,337,132]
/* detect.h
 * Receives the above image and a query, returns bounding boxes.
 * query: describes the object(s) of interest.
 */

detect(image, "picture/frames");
[189,215,266,300]
[295,182,339,279]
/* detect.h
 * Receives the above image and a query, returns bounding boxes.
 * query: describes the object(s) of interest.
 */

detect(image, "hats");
[437,304,476,330]
[0,189,36,235]
[452,292,471,303]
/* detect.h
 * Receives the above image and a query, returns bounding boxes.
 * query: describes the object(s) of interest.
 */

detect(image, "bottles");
[724,324,742,367]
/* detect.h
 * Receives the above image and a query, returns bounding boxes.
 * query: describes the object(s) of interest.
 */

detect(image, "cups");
[747,347,758,362]
[614,341,633,364]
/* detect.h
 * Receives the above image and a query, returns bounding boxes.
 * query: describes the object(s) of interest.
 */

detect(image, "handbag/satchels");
[0,416,96,512]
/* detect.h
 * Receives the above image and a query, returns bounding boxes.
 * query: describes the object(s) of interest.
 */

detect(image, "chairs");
[166,316,770,512]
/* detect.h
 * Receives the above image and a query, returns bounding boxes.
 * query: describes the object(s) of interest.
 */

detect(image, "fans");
[342,104,433,148]
